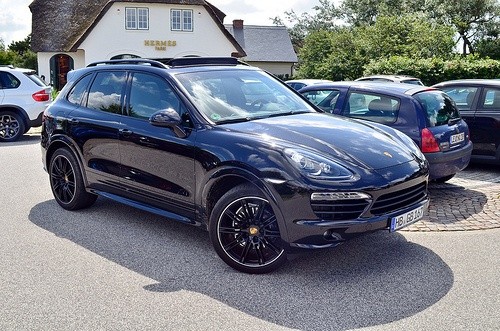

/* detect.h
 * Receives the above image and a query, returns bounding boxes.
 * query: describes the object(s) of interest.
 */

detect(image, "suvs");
[0,64,53,142]
[295,80,473,184]
[349,76,425,113]
[284,79,338,107]
[42,56,429,275]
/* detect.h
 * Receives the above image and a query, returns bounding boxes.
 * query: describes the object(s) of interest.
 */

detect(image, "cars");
[429,79,500,168]
